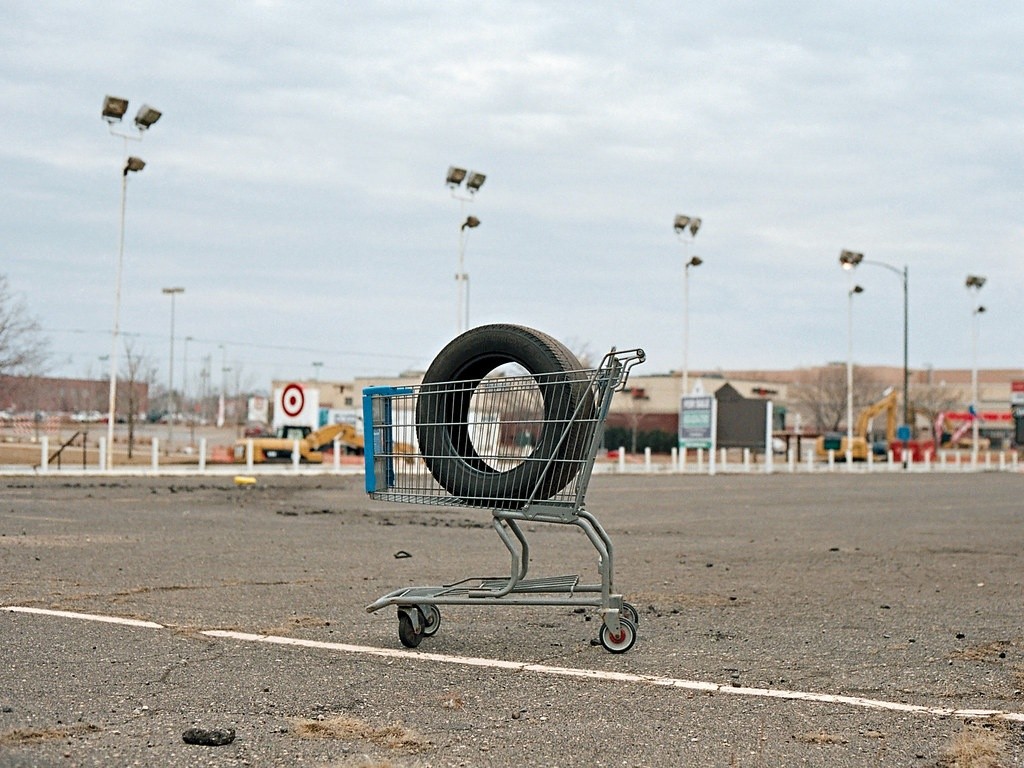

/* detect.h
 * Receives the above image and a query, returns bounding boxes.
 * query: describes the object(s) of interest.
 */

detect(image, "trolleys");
[362,346,649,655]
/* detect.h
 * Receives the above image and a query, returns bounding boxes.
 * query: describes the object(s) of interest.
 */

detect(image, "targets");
[281,384,304,416]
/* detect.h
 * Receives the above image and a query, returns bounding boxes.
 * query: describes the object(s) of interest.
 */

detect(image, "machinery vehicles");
[817,393,991,464]
[228,422,421,469]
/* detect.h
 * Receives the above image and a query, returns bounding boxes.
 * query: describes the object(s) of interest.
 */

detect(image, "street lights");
[846,282,865,466]
[964,274,987,462]
[839,248,915,449]
[98,95,162,461]
[161,286,185,457]
[446,163,485,335]
[673,212,704,400]
